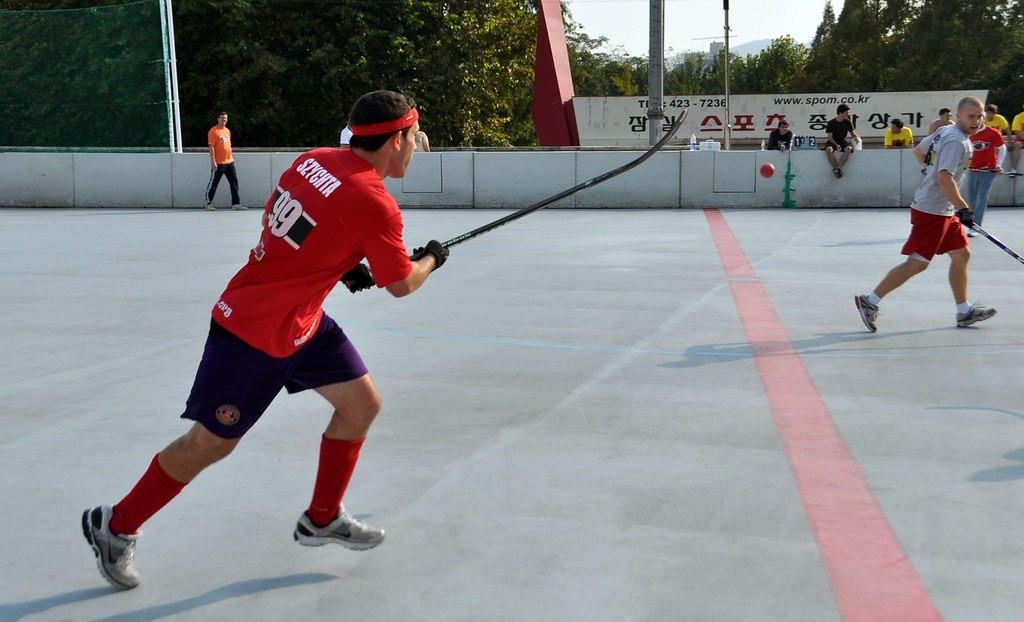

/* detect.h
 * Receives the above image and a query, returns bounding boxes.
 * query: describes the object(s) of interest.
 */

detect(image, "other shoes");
[833,168,843,178]
[1010,170,1017,178]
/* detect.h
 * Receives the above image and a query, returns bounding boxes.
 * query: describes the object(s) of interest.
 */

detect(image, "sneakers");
[855,294,878,332]
[293,504,385,551]
[203,202,216,210]
[232,202,248,210]
[967,226,978,238]
[81,506,140,589]
[956,300,996,327]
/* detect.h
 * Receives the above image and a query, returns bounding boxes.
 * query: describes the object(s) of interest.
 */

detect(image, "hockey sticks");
[955,210,1024,264]
[965,168,1023,178]
[346,106,689,289]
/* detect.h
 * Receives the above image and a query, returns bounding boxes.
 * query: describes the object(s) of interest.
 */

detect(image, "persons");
[964,112,1006,237]
[204,111,249,212]
[825,103,859,179]
[928,107,954,136]
[884,118,914,149]
[983,105,1013,152]
[767,120,793,152]
[1007,112,1024,177]
[81,91,449,592]
[854,96,997,333]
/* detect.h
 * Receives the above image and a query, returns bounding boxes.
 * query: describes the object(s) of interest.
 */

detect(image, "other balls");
[760,163,775,178]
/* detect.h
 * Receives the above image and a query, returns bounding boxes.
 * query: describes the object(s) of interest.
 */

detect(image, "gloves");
[412,240,450,271]
[340,262,376,293]
[957,208,974,228]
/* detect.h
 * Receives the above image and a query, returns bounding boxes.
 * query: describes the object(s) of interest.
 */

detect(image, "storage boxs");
[699,139,721,151]
